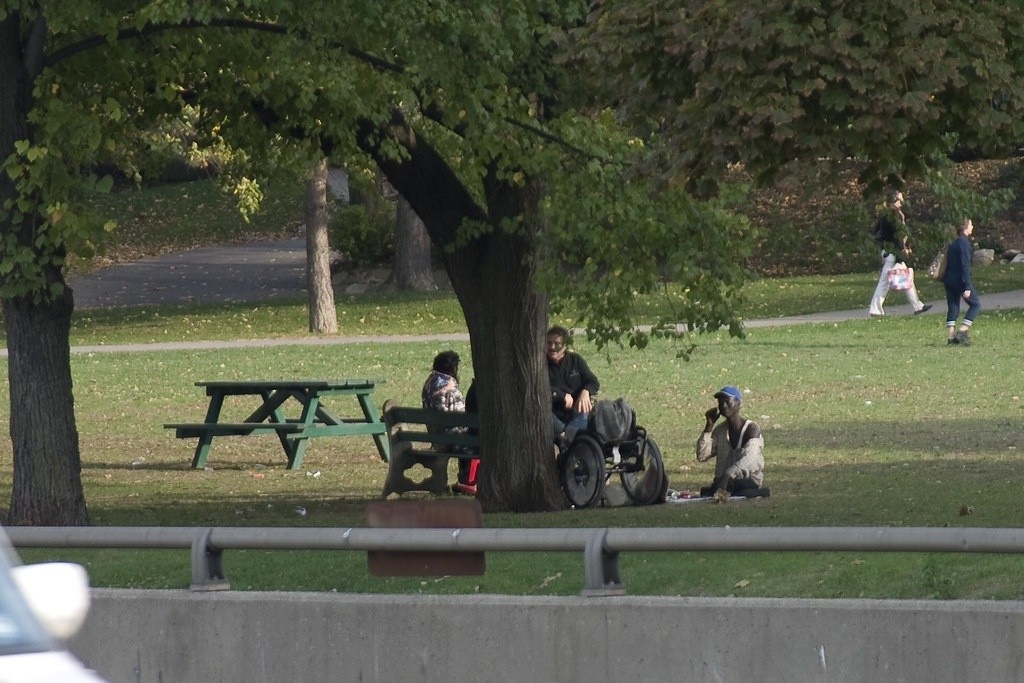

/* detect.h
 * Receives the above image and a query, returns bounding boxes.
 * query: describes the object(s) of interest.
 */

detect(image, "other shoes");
[454,481,478,495]
[947,337,956,347]
[914,304,933,315]
[871,313,890,317]
[954,330,973,346]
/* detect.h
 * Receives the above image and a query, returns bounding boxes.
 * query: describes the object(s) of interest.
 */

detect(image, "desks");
[192,378,390,470]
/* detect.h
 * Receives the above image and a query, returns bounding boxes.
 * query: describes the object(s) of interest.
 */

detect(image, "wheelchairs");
[559,398,664,509]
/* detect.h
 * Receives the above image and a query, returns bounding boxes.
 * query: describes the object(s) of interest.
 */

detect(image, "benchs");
[379,399,482,498]
[267,417,384,424]
[163,422,315,438]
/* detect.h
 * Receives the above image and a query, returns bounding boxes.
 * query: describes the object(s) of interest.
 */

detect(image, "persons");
[545,325,599,446]
[944,215,980,345]
[422,351,477,495]
[869,190,933,317]
[696,386,765,501]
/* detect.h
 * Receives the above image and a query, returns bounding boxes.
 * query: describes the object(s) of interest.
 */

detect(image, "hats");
[714,386,741,401]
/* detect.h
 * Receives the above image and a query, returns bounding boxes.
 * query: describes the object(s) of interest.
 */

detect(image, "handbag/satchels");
[885,261,914,291]
[927,242,950,281]
